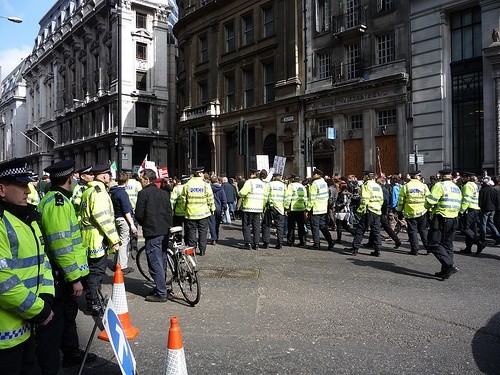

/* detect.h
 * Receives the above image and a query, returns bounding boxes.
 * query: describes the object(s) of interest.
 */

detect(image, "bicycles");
[136,226,201,306]
[379,213,430,242]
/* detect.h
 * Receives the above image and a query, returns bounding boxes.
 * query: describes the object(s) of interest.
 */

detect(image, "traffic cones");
[97,264,139,342]
[164,315,188,375]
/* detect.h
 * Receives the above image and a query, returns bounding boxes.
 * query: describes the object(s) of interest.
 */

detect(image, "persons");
[302,172,362,245]
[37,160,97,370]
[261,174,288,249]
[135,169,173,302]
[78,164,120,315]
[160,167,245,256]
[304,168,335,249]
[238,169,266,250]
[424,169,462,279]
[73,166,95,211]
[397,171,432,255]
[283,174,308,247]
[0,159,56,375]
[110,171,142,276]
[422,170,500,255]
[362,174,411,249]
[72,168,80,190]
[28,175,50,205]
[344,171,384,255]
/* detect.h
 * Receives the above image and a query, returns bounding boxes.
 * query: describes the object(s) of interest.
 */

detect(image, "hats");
[193,166,204,173]
[248,167,323,179]
[44,159,76,179]
[0,157,36,182]
[77,164,92,175]
[90,164,112,175]
[361,169,474,180]
[180,176,190,180]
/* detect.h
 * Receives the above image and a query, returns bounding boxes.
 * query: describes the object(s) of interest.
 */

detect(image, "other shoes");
[145,293,168,303]
[435,271,441,278]
[261,243,268,249]
[297,241,306,246]
[200,250,205,256]
[334,238,341,244]
[327,240,334,249]
[441,264,459,281]
[283,243,293,246]
[408,251,418,256]
[310,246,320,249]
[474,243,486,254]
[209,240,216,245]
[494,240,500,246]
[344,247,359,255]
[275,244,282,249]
[86,297,106,315]
[458,246,471,253]
[371,250,380,255]
[394,241,401,249]
[242,243,251,250]
[253,244,258,250]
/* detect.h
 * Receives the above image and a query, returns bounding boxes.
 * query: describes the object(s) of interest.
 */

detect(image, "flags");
[138,156,147,177]
[110,161,117,179]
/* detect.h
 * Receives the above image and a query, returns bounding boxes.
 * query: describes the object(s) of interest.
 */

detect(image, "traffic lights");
[301,139,305,155]
